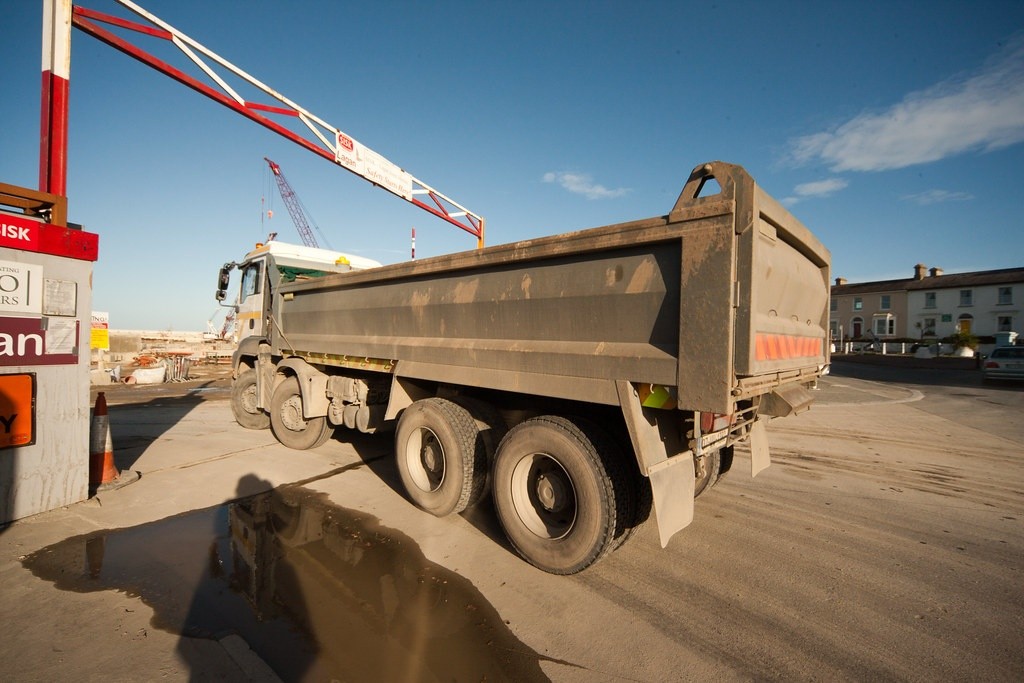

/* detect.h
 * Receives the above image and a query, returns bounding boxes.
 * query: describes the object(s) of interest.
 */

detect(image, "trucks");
[215,159,831,578]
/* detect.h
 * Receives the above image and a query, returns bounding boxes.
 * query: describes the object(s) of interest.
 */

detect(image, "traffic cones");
[90,390,120,485]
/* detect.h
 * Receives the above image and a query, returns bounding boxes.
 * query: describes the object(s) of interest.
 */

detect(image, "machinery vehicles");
[263,157,320,248]
[202,310,236,340]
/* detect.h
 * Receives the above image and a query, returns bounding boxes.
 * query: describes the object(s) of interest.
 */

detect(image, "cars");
[983,345,1024,387]
